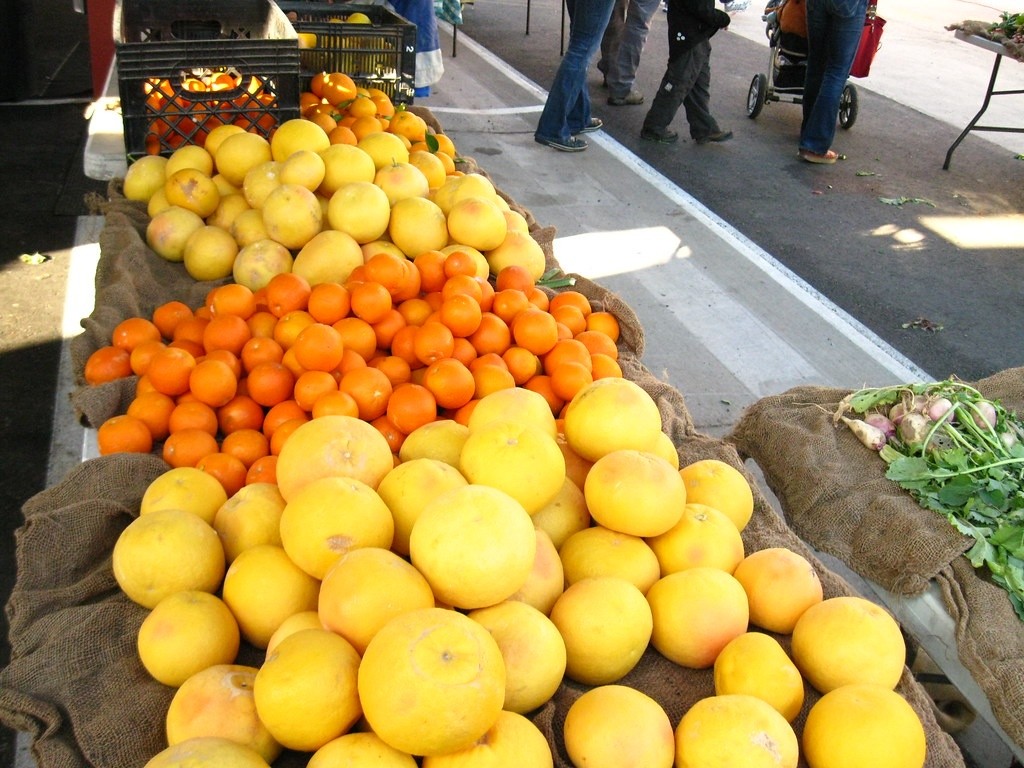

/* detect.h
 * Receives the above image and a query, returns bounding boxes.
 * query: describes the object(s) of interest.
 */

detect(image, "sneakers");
[534,135,588,152]
[696,130,733,145]
[603,77,608,87]
[608,90,644,107]
[575,118,603,135]
[641,130,679,144]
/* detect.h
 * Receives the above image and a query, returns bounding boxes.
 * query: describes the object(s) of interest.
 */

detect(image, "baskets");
[112,0,301,168]
[276,2,415,107]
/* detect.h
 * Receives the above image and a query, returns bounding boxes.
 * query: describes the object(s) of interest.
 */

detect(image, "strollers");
[747,0,888,130]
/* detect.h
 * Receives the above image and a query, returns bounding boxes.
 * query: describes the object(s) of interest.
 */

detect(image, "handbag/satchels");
[850,0,886,78]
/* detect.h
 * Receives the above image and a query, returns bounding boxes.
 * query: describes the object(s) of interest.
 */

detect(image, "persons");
[532,0,615,152]
[662,0,750,17]
[639,0,735,146]
[795,0,868,164]
[596,0,661,105]
[384,0,446,99]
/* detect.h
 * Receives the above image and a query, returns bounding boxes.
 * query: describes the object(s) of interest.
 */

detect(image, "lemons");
[293,12,396,99]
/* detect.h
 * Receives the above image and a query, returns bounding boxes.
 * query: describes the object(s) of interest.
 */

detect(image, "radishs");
[790,387,996,449]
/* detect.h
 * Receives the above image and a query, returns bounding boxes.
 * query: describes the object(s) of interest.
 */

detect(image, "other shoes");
[798,148,837,164]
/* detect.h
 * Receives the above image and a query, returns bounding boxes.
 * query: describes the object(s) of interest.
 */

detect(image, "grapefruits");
[104,376,926,768]
[119,117,545,289]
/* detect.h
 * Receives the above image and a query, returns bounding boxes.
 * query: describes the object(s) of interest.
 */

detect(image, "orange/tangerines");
[84,243,622,493]
[143,71,463,176]
